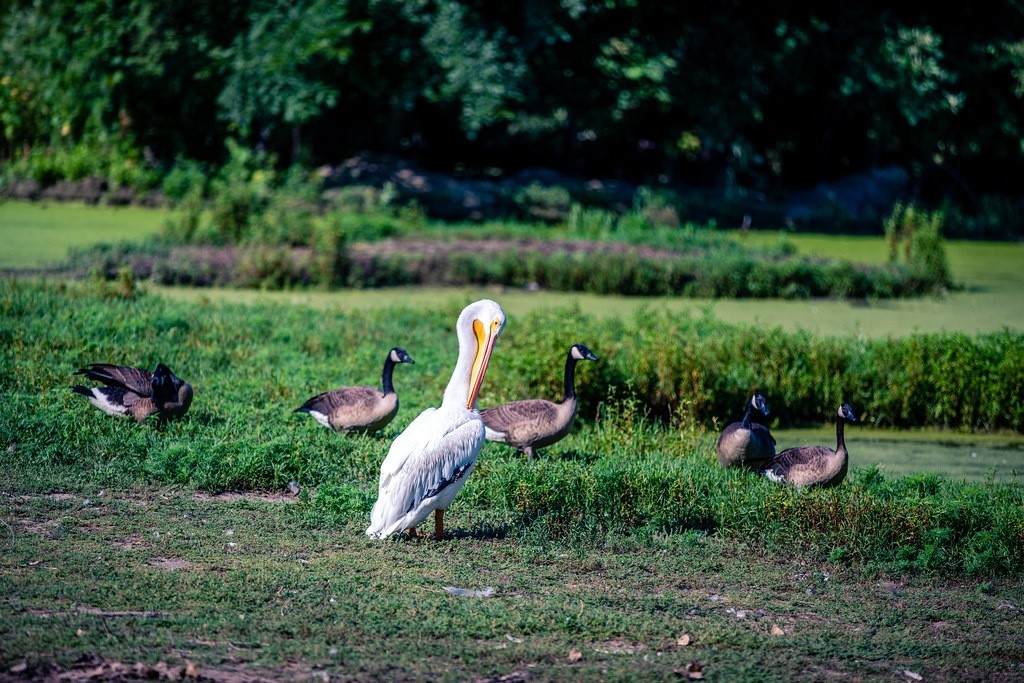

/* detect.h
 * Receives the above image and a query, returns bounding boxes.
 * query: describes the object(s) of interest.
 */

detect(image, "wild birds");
[67,343,856,497]
[364,297,506,541]
